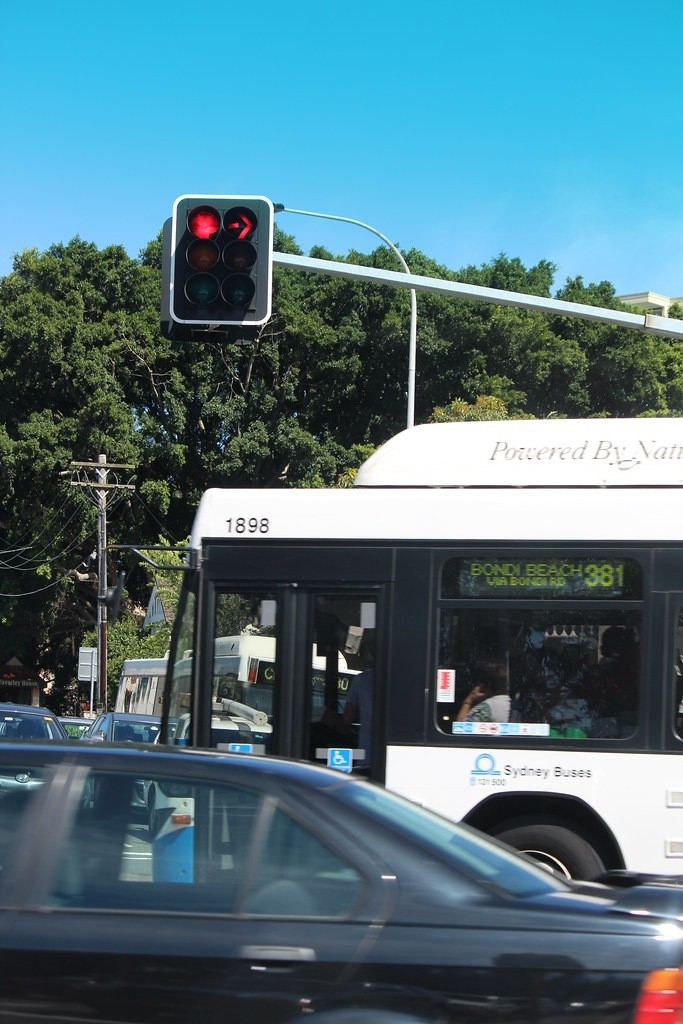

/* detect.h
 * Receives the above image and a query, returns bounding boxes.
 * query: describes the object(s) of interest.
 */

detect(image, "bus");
[114,636,363,729]
[76,417,683,889]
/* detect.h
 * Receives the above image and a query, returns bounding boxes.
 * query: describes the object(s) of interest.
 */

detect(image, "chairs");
[46,824,106,908]
[244,879,315,917]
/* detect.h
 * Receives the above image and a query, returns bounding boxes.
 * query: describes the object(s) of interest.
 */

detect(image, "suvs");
[143,710,273,843]
[0,702,70,801]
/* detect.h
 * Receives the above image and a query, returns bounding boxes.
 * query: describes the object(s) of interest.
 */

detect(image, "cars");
[0,743,682,1024]
[80,715,178,817]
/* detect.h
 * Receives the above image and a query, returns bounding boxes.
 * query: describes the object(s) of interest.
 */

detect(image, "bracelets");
[462,700,473,706]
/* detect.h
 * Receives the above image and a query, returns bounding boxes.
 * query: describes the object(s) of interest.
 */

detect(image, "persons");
[344,632,376,783]
[442,625,638,739]
[456,669,511,723]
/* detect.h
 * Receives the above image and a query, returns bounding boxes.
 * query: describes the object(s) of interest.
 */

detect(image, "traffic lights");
[171,195,272,327]
